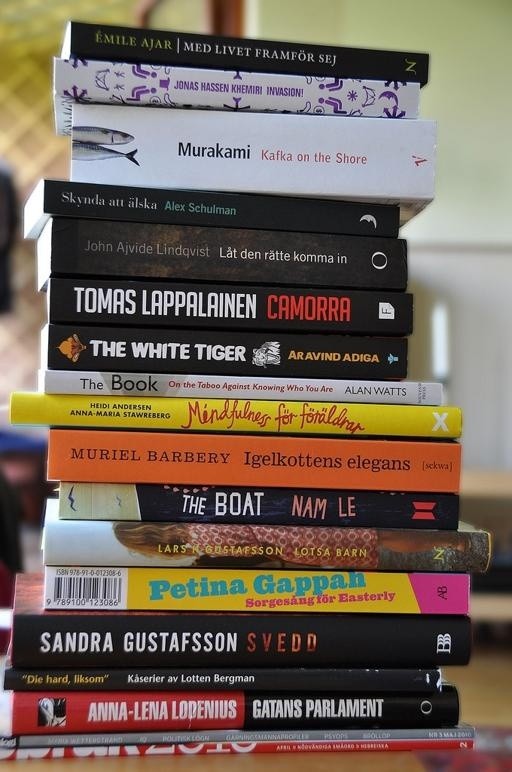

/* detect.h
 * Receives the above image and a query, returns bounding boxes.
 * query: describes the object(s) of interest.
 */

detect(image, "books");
[1,12,492,759]
